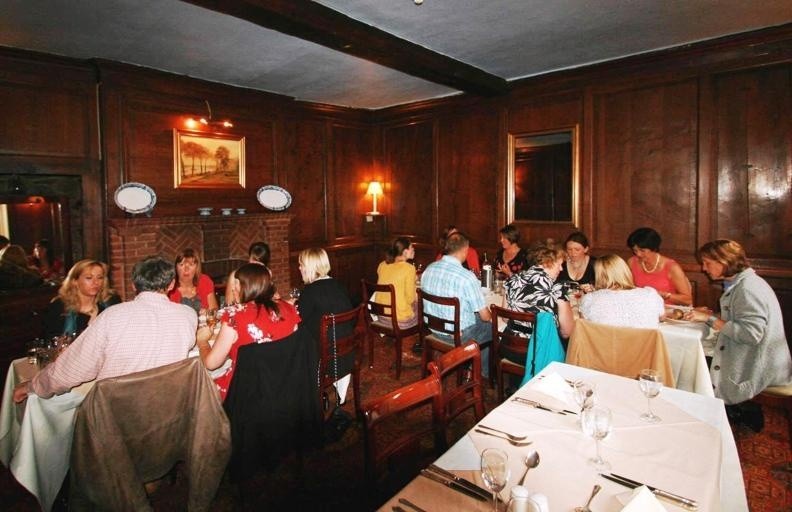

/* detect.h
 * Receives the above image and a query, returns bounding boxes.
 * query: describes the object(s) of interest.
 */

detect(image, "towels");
[516,372,582,414]
[615,485,670,512]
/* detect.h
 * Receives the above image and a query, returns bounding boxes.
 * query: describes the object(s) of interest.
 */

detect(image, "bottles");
[481,270,493,290]
[206,303,217,328]
[482,251,491,270]
[28,333,76,370]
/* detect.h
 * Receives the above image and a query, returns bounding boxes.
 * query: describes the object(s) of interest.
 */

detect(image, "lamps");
[363,181,384,216]
[184,100,234,131]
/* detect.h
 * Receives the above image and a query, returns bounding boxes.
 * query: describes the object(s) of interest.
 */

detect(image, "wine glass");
[481,369,662,512]
[288,287,303,299]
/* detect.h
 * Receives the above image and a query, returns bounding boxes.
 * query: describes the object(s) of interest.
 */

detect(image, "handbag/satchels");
[323,407,353,444]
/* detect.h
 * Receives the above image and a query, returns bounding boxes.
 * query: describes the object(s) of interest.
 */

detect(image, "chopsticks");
[599,472,699,511]
[427,464,503,505]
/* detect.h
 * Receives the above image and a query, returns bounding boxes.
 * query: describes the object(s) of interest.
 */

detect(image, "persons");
[42,260,123,344]
[13,253,199,403]
[576,255,667,330]
[685,236,792,431]
[169,241,281,314]
[196,264,302,403]
[495,226,597,365]
[1,232,62,292]
[420,225,494,382]
[375,237,420,363]
[625,227,692,305]
[294,246,356,378]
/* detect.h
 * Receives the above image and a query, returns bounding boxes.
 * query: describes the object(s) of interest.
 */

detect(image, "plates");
[665,304,694,324]
[114,182,157,214]
[256,184,292,211]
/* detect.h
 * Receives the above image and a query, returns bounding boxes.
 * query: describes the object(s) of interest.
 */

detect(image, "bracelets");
[664,291,672,301]
[705,315,718,327]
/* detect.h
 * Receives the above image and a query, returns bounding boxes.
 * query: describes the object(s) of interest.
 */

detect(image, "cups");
[494,270,504,294]
[198,207,247,216]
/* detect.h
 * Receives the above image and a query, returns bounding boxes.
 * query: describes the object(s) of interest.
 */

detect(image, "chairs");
[0,287,61,406]
[571,320,663,386]
[317,280,485,503]
[53,356,200,509]
[752,378,791,412]
[489,304,556,402]
[224,326,317,480]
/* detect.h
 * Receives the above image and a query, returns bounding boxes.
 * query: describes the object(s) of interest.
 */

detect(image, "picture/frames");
[173,127,246,190]
[506,123,580,230]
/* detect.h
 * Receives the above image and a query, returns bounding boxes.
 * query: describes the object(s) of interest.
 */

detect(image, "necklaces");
[639,254,662,274]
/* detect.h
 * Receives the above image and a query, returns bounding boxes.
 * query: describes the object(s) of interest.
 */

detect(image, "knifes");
[420,468,488,502]
